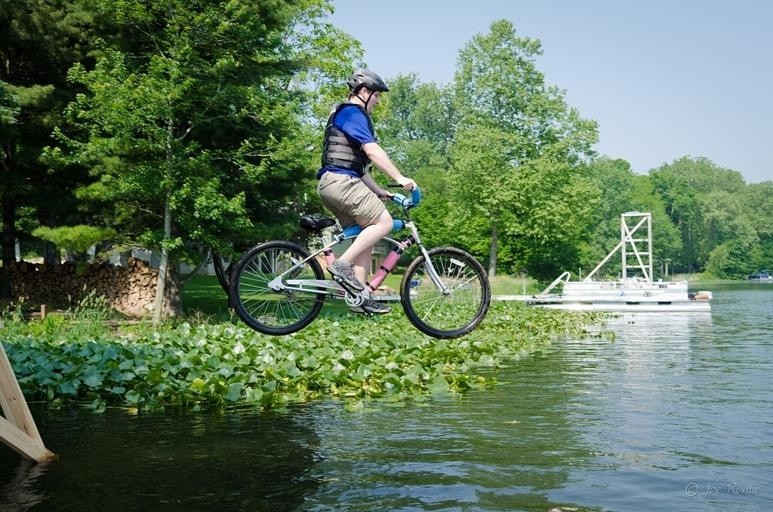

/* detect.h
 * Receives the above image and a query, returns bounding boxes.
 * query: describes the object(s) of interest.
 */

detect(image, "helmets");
[347,68,390,92]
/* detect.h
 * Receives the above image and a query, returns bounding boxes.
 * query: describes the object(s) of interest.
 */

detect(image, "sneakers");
[350,294,392,313]
[326,260,364,291]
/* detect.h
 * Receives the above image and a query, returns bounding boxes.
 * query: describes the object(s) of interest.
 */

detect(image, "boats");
[526,219,710,314]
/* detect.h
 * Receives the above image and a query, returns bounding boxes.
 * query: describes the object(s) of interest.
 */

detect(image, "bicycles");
[229,184,490,338]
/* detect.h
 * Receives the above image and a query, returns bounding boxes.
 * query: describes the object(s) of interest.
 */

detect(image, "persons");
[317,70,418,317]
